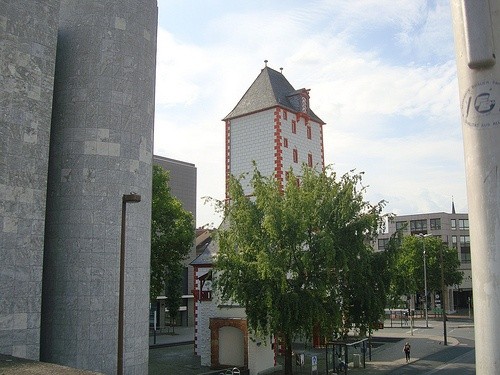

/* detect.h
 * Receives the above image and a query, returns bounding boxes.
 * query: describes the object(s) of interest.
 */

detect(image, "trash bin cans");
[353,354,360,368]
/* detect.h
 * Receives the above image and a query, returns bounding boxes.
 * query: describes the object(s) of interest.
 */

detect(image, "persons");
[404,313,408,325]
[404,342,410,362]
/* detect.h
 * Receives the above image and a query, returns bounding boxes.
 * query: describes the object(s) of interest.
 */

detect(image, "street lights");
[117,193,142,375]
[418,232,428,327]
